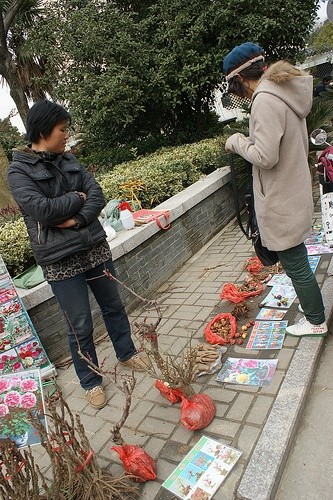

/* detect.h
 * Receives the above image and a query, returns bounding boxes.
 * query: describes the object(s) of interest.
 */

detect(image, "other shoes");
[118,354,150,372]
[84,385,106,409]
[285,316,328,338]
[297,304,305,313]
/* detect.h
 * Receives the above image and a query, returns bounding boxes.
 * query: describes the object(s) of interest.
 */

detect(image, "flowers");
[0,301,47,374]
[0,375,38,438]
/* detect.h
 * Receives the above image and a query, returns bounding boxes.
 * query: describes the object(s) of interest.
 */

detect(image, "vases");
[9,432,28,447]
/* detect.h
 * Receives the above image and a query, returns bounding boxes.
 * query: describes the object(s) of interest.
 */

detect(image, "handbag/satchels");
[228,152,278,267]
[131,208,172,231]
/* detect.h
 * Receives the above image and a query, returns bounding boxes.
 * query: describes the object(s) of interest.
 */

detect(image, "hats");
[222,42,264,82]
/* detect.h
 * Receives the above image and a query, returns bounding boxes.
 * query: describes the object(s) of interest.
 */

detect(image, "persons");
[224,41,328,337]
[6,101,151,408]
